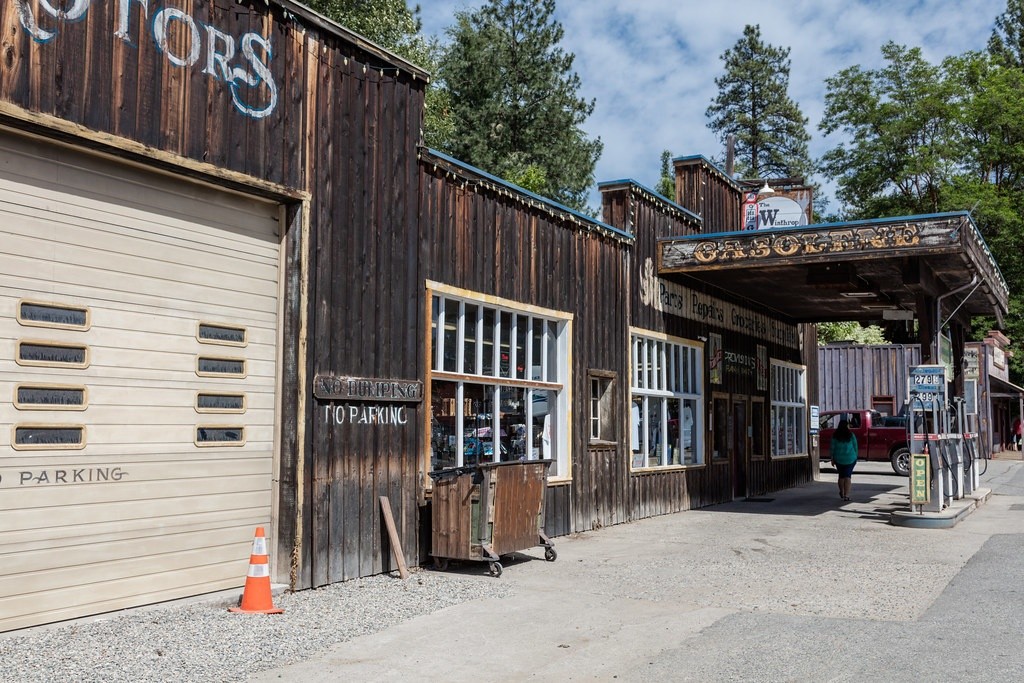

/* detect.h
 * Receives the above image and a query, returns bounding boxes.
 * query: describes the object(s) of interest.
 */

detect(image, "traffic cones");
[228,526,285,615]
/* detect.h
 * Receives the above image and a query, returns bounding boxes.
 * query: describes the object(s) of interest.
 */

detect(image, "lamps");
[757,178,774,195]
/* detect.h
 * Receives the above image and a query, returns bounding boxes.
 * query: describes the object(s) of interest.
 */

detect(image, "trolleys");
[426,458,557,578]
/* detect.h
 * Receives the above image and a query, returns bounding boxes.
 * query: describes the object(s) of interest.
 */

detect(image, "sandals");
[839,492,843,498]
[844,497,851,501]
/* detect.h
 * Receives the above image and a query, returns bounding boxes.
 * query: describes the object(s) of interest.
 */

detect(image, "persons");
[1011,415,1022,451]
[829,420,859,501]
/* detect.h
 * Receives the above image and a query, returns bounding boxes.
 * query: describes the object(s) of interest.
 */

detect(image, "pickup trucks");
[818,410,911,476]
